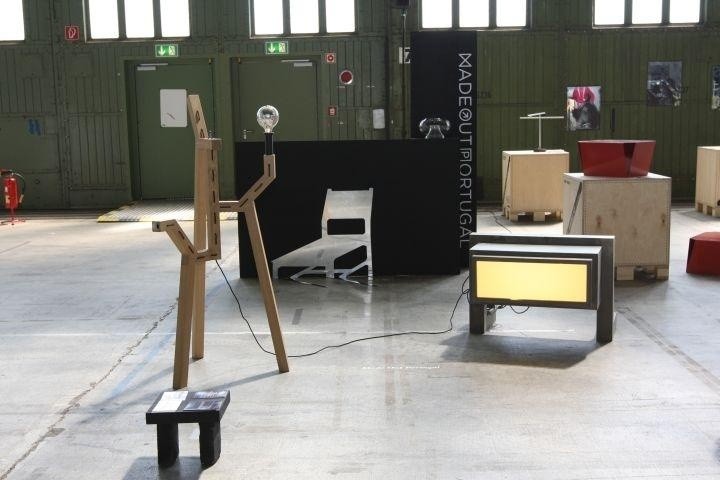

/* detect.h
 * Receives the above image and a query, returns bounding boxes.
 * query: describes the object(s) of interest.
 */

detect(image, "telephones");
[419,117,451,139]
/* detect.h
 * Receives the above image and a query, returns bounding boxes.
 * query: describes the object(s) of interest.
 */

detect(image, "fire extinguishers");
[0,170,26,208]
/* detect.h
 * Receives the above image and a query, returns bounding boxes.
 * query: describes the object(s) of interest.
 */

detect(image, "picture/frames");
[709,64,720,111]
[645,59,684,107]
[565,84,604,130]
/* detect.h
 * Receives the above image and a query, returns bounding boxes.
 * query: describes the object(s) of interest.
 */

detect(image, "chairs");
[270,187,374,279]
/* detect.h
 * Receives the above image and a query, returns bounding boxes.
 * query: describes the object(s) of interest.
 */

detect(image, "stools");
[145,388,231,469]
